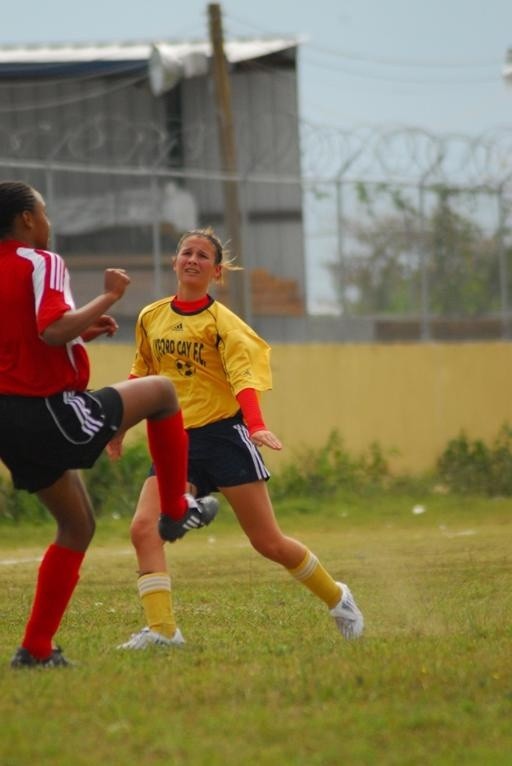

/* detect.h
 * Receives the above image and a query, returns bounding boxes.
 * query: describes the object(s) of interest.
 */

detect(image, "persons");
[0,181,219,669]
[104,227,364,653]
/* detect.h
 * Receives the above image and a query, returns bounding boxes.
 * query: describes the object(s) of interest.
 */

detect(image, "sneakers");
[12,649,68,668]
[158,496,218,543]
[329,583,363,639]
[118,627,184,650]
